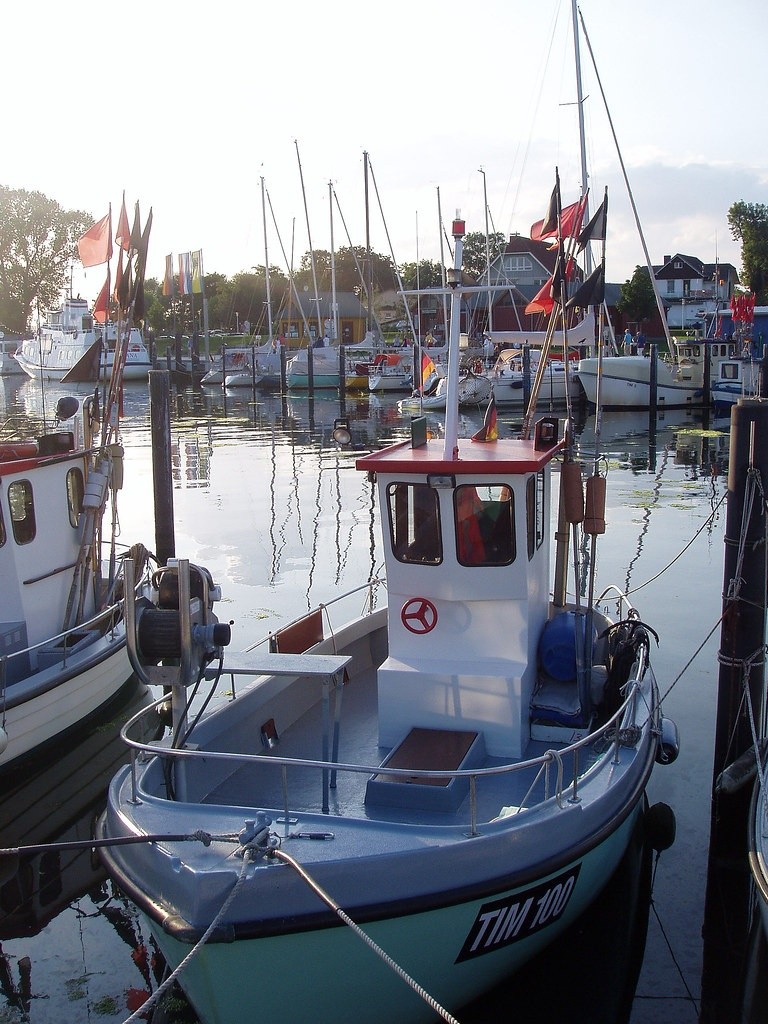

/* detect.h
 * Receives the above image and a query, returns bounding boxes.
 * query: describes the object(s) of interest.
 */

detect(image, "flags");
[524,177,607,316]
[79,193,205,325]
[421,350,435,383]
[471,390,498,442]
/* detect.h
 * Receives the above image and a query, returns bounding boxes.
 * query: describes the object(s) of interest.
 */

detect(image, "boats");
[0,338,30,378]
[0,391,156,773]
[12,265,154,384]
[92,213,679,1024]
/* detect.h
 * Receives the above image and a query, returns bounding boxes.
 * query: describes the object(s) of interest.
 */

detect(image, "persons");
[393,332,436,346]
[621,329,646,356]
[151,328,351,359]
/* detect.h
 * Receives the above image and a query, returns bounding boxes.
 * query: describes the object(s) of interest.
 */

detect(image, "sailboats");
[193,0,768,414]
